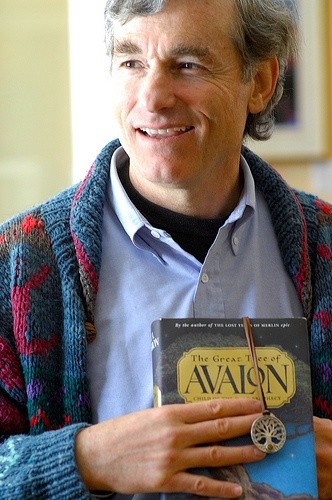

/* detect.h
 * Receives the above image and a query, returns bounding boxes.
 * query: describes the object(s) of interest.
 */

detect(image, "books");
[151,315,319,500]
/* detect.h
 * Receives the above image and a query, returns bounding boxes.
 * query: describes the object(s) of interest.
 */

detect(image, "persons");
[1,0,332,498]
